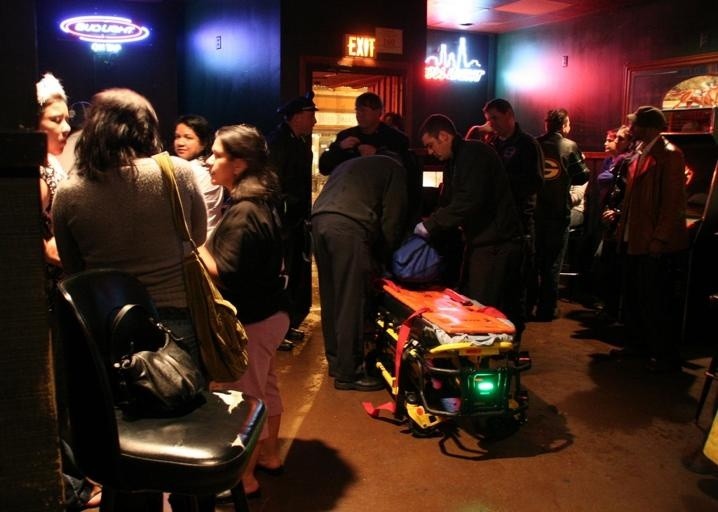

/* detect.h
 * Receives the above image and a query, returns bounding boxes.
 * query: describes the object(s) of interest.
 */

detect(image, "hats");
[276,90,319,119]
[628,106,666,130]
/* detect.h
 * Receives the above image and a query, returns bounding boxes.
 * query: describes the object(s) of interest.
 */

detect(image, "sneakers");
[218,482,262,503]
[285,328,304,338]
[257,457,282,478]
[278,336,292,350]
[81,480,102,506]
[536,308,562,318]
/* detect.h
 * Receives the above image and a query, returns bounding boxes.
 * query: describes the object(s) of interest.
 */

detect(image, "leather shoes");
[328,370,384,391]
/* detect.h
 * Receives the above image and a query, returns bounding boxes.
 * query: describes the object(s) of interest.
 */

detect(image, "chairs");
[52,266,268,512]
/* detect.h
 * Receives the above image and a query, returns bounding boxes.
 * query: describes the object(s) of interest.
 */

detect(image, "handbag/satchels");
[111,331,206,420]
[182,250,247,383]
[392,233,442,285]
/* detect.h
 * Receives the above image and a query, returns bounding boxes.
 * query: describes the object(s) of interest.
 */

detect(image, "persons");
[317,92,690,378]
[263,89,321,352]
[172,113,225,264]
[39,71,76,214]
[51,85,215,510]
[194,123,293,506]
[305,150,409,390]
